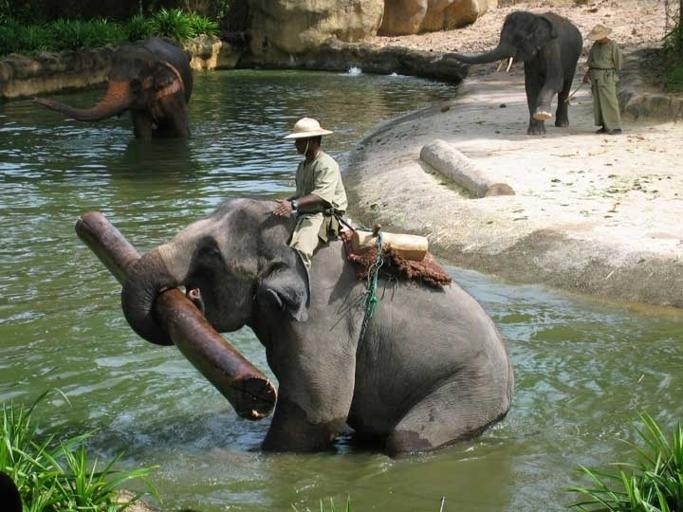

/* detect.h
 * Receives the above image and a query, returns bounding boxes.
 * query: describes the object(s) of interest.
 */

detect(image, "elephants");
[442,10,583,137]
[30,33,195,143]
[119,194,515,461]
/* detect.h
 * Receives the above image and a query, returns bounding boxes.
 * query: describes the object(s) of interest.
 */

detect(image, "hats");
[284,117,333,139]
[587,24,612,41]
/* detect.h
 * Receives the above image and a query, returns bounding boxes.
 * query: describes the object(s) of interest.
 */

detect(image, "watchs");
[291,199,298,210]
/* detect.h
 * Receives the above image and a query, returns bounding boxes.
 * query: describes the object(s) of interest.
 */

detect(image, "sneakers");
[264,289,286,314]
[609,129,621,134]
[596,127,608,133]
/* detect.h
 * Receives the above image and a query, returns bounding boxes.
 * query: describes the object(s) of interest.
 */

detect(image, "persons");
[584,25,623,135]
[261,117,349,309]
